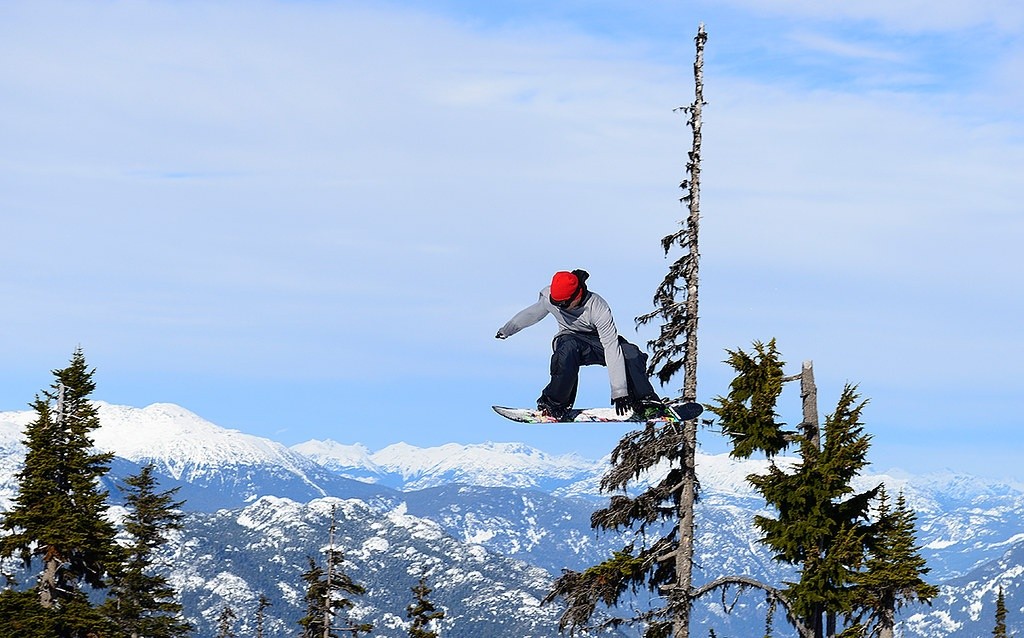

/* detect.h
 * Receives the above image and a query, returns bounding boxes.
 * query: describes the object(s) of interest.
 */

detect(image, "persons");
[495,268,665,422]
[42,546,69,588]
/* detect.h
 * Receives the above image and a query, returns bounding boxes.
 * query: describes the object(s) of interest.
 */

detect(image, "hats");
[550,272,579,301]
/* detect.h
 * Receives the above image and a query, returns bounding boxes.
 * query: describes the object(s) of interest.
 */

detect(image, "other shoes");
[638,394,665,418]
[541,408,558,419]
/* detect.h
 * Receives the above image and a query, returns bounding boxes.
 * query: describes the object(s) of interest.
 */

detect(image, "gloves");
[611,397,631,416]
[495,331,508,339]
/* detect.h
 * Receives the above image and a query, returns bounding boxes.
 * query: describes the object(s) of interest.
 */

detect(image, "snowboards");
[492,402,704,424]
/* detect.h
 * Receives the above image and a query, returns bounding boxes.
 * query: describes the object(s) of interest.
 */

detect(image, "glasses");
[550,297,568,309]
